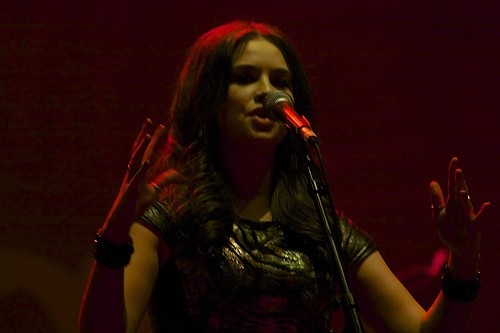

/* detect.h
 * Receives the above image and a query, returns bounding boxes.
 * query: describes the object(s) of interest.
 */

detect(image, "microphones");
[264,89,319,146]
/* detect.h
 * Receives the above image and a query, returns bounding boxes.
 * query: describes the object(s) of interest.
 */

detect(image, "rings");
[431,203,446,210]
[127,162,136,175]
[460,195,471,204]
[77,19,490,333]
[137,171,145,179]
[152,181,162,191]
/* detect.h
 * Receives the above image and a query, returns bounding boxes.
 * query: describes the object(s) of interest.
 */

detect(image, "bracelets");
[442,264,481,303]
[93,227,135,270]
